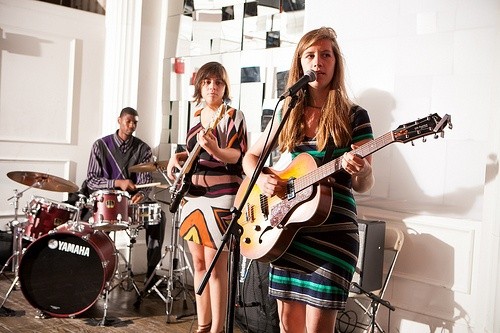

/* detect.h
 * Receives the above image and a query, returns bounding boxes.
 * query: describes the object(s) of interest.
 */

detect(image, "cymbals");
[127,161,168,173]
[7,170,79,193]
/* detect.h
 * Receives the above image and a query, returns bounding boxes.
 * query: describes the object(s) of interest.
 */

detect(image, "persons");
[243,27,373,333]
[86,107,166,288]
[167,62,247,333]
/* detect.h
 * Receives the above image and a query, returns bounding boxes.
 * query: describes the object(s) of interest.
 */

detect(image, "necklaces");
[309,104,322,110]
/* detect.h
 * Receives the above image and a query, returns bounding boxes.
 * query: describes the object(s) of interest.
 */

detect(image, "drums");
[87,189,131,231]
[128,201,161,225]
[22,195,79,241]
[18,220,118,318]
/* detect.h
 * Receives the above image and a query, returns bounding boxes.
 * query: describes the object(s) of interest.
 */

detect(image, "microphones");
[280,69,318,101]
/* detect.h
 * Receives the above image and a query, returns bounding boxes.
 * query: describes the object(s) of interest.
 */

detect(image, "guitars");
[232,113,453,263]
[168,104,228,212]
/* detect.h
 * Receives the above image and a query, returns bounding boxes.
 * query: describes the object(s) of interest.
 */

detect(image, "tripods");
[0,181,40,312]
[103,192,194,325]
